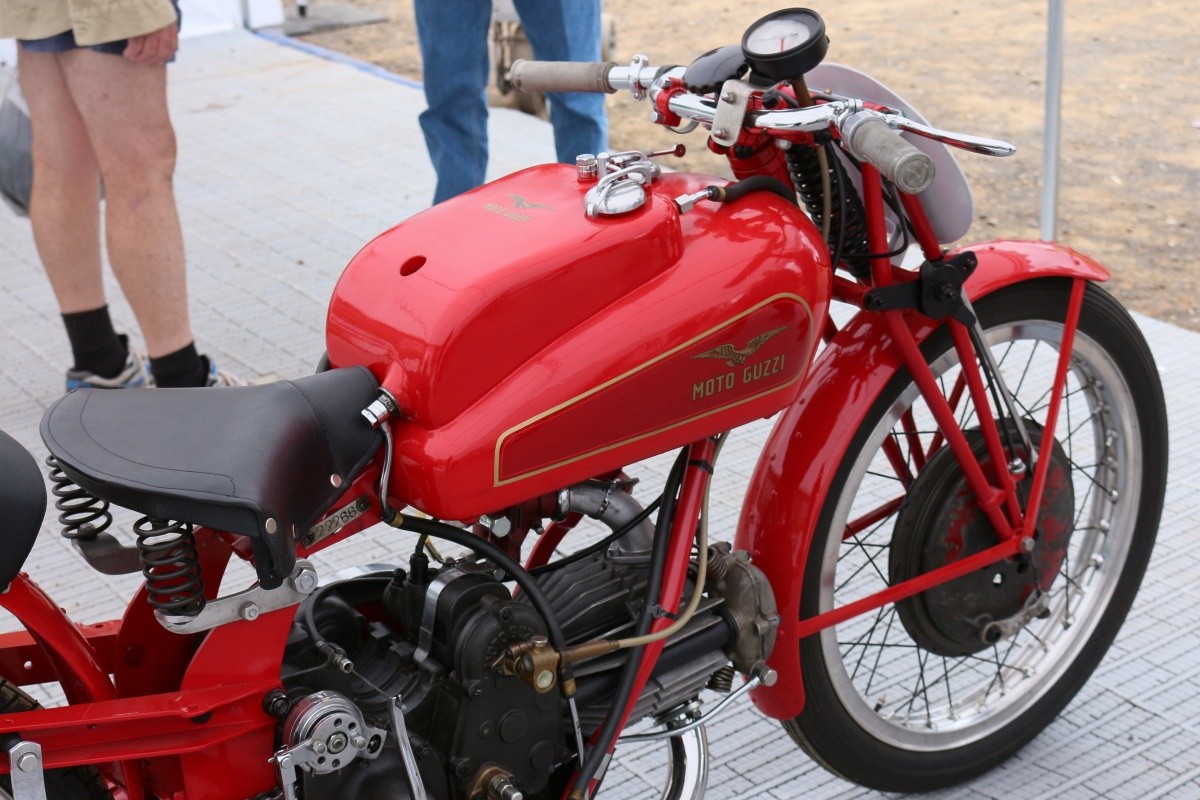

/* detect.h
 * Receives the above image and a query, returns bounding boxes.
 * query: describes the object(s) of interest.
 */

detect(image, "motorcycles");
[0,5,1171,800]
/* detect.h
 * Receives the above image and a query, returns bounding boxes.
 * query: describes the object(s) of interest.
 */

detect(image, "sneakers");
[200,354,280,387]
[66,334,156,393]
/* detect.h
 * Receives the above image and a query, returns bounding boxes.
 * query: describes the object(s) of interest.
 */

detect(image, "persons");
[0,0,277,392]
[413,1,609,207]
